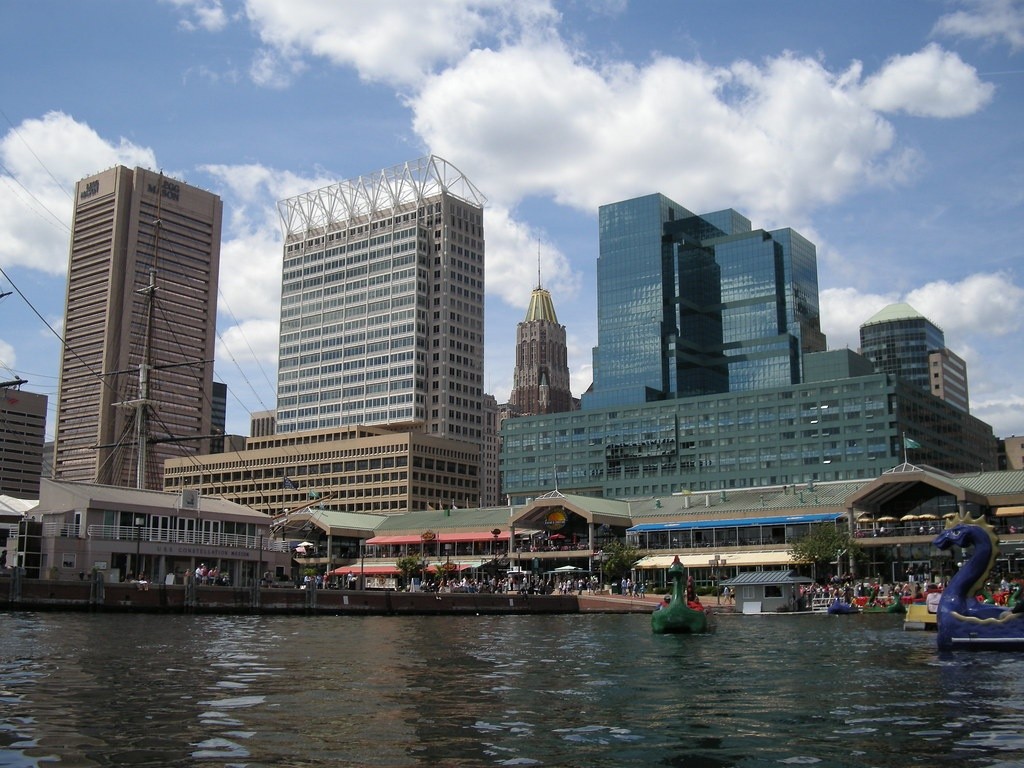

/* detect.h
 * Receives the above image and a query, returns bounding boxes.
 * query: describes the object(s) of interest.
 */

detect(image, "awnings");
[365,531,520,544]
[326,559,492,574]
[997,507,1024,515]
[626,504,846,533]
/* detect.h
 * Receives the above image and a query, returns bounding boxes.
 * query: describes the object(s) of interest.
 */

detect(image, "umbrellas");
[859,513,957,522]
[297,542,314,546]
[534,533,565,539]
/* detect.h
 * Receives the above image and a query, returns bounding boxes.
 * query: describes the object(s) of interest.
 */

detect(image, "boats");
[651,555,707,635]
[826,510,1024,645]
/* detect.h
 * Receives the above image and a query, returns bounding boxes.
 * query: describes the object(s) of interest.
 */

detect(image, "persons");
[799,562,951,607]
[856,525,935,538]
[185,563,232,586]
[723,586,734,604]
[126,570,152,590]
[1000,576,1009,591]
[420,576,513,594]
[261,570,357,589]
[523,576,645,599]
[1009,523,1016,533]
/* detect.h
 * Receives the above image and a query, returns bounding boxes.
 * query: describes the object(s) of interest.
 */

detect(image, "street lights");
[256,528,265,585]
[444,543,452,586]
[358,537,366,590]
[597,549,605,595]
[709,554,728,606]
[516,546,524,595]
[135,513,145,581]
